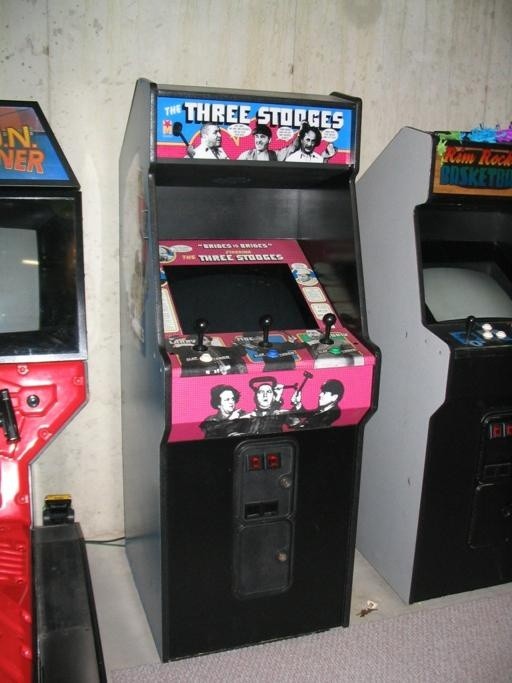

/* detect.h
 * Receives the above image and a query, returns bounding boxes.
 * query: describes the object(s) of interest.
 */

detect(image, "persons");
[202,375,343,443]
[186,121,337,164]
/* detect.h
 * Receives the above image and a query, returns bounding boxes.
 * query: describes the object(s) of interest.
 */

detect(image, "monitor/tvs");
[423,267,512,322]
[0,227,41,333]
[163,264,319,334]
